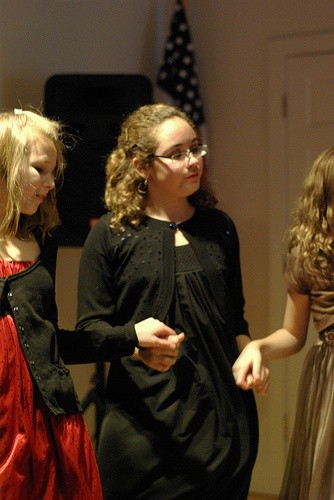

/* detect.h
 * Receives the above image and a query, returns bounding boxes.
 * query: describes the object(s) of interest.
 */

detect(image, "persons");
[76,102,272,500]
[232,148,334,500]
[0,107,176,500]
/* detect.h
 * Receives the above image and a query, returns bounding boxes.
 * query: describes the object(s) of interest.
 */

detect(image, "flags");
[150,0,209,165]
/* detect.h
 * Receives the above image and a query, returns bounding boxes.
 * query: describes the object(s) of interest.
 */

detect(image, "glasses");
[152,143,209,166]
